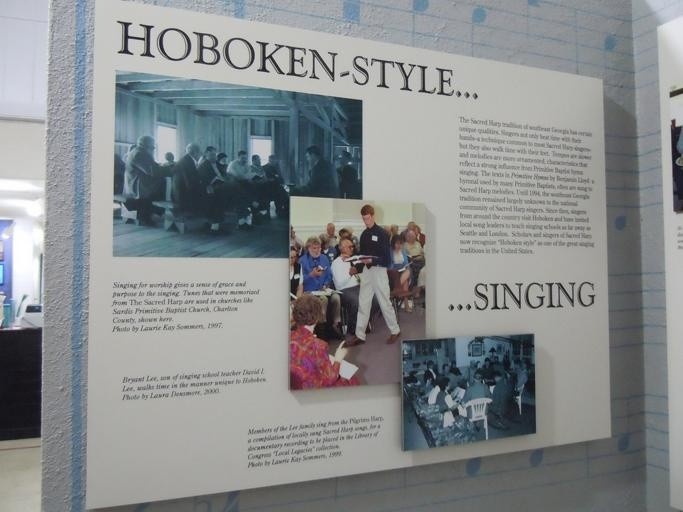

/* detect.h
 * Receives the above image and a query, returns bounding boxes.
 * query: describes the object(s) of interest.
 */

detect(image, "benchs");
[113,195,200,234]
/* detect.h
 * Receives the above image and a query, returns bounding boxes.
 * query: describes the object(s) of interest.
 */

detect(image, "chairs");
[461,397,493,440]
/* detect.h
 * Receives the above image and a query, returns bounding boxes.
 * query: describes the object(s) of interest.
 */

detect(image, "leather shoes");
[348,325,356,334]
[343,335,365,347]
[386,333,400,344]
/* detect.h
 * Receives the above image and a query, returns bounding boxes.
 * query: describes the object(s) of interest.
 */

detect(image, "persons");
[289,220,424,391]
[114,130,359,238]
[421,355,532,435]
[341,204,400,346]
